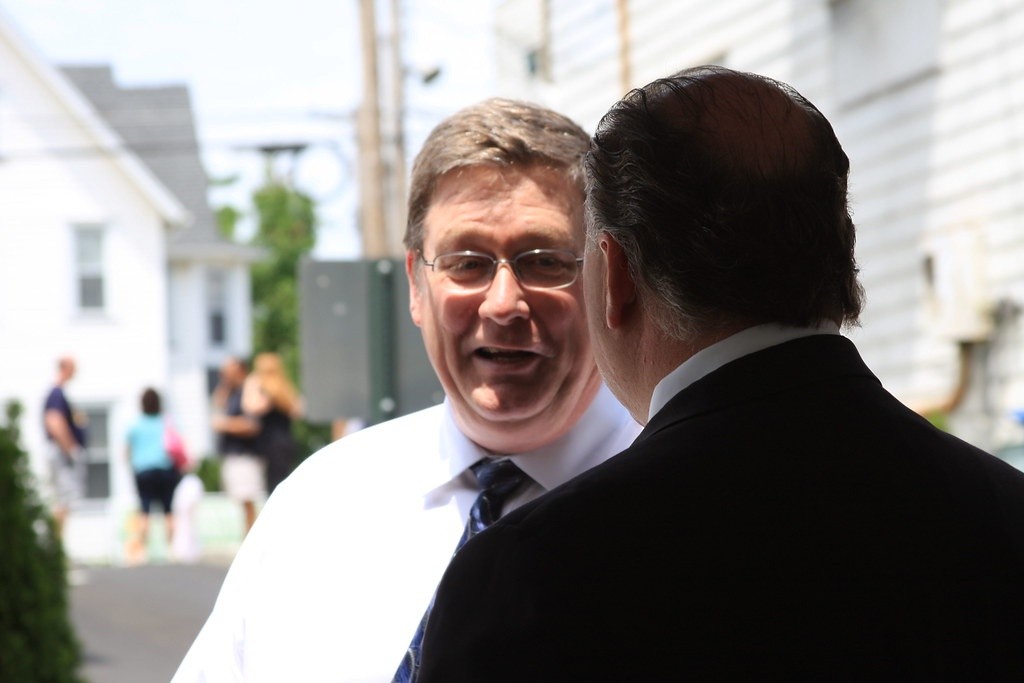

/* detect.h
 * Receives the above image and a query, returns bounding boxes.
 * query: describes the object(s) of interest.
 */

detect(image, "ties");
[390,456,535,683]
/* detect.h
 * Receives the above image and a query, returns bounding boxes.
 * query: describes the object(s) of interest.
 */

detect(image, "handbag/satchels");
[163,419,189,471]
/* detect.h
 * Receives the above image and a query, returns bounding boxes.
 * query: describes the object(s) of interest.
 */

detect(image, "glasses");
[416,248,584,291]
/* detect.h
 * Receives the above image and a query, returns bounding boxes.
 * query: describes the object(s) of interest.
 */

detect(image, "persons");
[119,387,186,561]
[208,351,306,536]
[41,354,90,569]
[416,63,1024,683]
[169,95,643,683]
